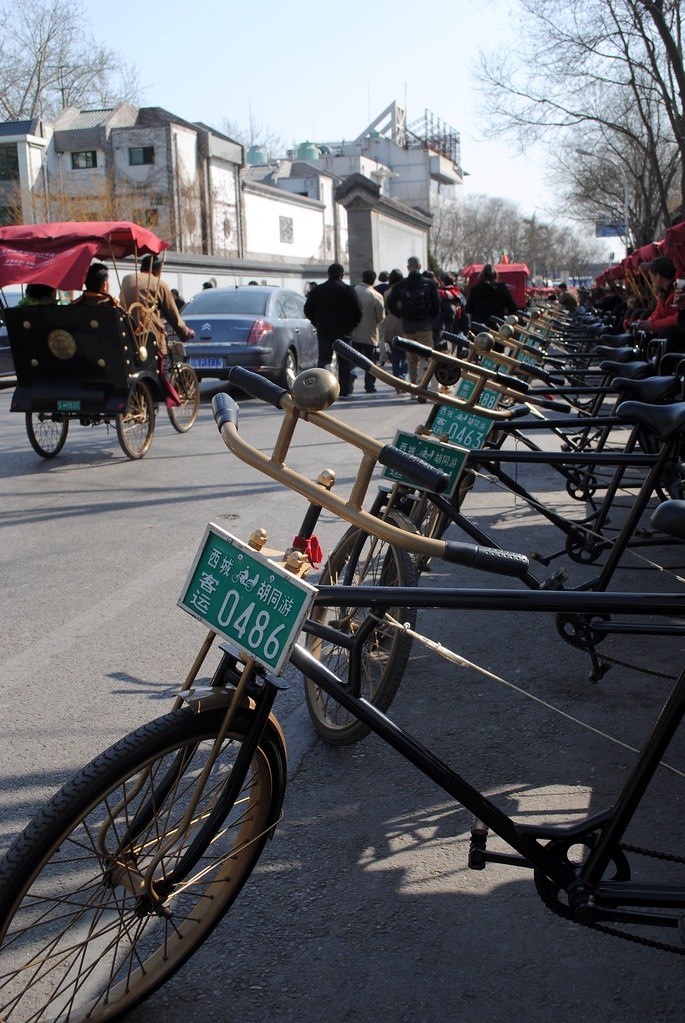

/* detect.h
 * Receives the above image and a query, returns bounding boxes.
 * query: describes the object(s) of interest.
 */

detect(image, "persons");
[119,255,195,379]
[304,257,520,397]
[548,283,577,313]
[162,289,185,320]
[579,257,685,350]
[67,263,136,331]
[202,282,213,289]
[19,284,61,305]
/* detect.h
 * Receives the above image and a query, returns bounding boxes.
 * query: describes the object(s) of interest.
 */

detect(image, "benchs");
[1,306,151,374]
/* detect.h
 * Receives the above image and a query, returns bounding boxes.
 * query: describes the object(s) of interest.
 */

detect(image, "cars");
[163,285,339,392]
[0,292,26,379]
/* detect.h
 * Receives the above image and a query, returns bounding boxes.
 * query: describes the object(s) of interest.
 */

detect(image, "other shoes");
[416,382,427,402]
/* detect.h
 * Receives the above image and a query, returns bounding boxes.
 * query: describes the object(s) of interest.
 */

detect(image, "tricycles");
[0,221,200,462]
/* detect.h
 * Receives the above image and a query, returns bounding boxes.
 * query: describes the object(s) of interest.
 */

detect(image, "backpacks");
[400,278,430,322]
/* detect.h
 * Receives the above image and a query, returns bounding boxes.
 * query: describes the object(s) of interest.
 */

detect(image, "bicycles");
[0,366,685,1023]
[303,335,684,746]
[384,223,685,590]
